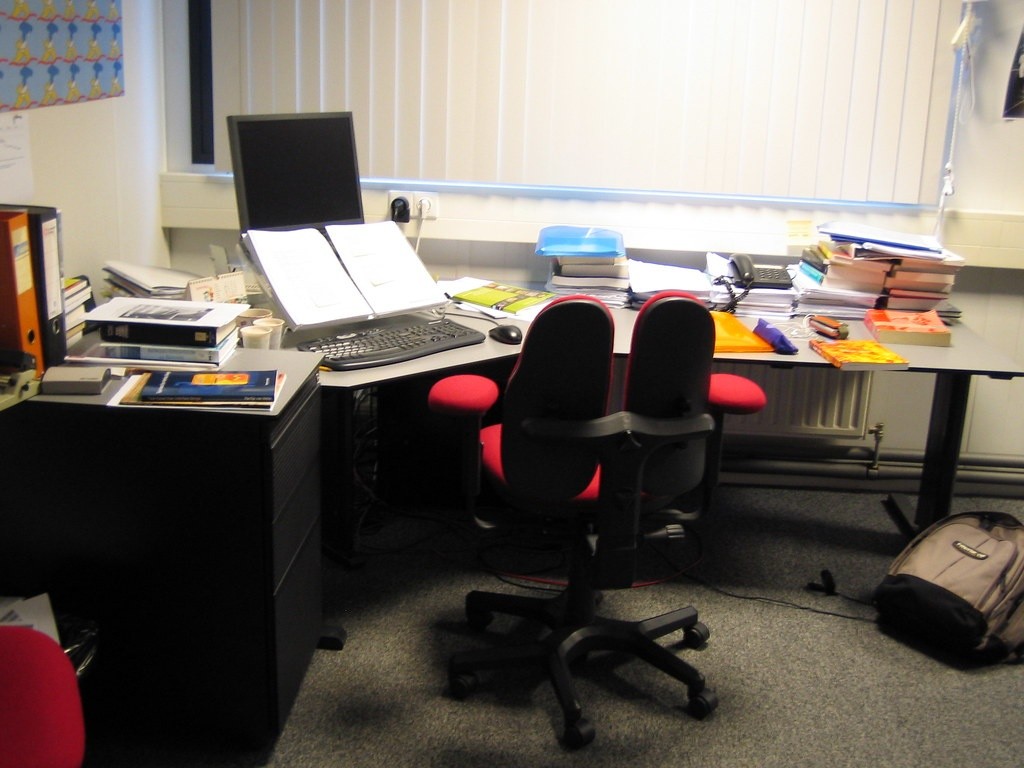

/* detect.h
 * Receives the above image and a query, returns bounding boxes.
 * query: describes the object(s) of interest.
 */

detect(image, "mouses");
[489,325,522,343]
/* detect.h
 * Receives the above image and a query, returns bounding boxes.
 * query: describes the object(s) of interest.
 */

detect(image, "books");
[235,219,450,333]
[438,226,963,352]
[809,338,910,370]
[0,204,299,414]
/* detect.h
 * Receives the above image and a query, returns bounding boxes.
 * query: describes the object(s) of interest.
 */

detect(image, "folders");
[0,203,69,380]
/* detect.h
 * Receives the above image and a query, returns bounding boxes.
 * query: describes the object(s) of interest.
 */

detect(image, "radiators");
[609,356,874,439]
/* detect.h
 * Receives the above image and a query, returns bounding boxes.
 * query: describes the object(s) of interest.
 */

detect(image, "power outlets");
[389,190,436,220]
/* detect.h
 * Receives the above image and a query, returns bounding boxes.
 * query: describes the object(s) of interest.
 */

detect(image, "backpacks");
[873,510,1024,669]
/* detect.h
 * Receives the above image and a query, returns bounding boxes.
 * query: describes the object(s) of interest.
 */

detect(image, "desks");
[317,280,1024,589]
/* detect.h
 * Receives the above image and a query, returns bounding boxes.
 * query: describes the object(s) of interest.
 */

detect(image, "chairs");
[426,296,769,749]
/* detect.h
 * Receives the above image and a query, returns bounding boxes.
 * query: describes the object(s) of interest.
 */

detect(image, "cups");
[240,326,271,350]
[238,308,273,327]
[251,317,285,350]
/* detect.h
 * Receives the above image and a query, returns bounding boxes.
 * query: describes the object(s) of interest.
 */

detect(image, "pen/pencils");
[319,366,335,373]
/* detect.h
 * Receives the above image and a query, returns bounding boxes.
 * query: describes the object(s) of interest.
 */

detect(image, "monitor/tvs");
[227,111,364,234]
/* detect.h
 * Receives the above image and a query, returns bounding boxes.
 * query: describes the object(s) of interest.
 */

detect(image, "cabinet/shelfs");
[1,360,345,768]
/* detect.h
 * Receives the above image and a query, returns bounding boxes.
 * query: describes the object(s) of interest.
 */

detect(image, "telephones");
[727,253,794,290]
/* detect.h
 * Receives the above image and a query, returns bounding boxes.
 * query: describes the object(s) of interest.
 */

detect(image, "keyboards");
[296,317,485,371]
